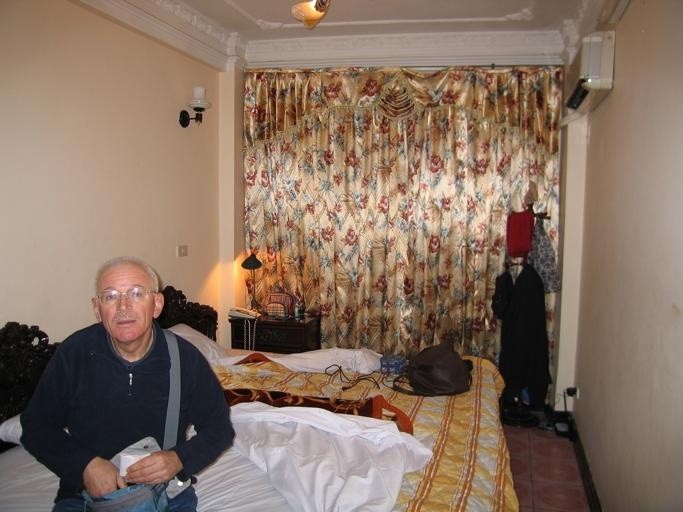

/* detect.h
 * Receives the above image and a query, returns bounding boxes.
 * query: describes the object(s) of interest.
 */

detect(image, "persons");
[20,257,236,512]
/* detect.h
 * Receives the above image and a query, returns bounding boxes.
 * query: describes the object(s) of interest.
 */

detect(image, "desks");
[232,314,321,353]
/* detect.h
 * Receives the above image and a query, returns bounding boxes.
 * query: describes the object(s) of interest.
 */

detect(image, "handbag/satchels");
[381,354,406,374]
[529,223,560,294]
[82,482,170,512]
[406,344,471,397]
[264,293,293,318]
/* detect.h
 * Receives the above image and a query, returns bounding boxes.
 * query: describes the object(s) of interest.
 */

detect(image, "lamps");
[241,252,263,315]
[291,1,331,32]
[178,85,212,128]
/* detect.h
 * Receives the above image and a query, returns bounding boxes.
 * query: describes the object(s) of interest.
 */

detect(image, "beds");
[1,284,521,512]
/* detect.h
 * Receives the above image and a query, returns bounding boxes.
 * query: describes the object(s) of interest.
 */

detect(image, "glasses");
[95,285,158,308]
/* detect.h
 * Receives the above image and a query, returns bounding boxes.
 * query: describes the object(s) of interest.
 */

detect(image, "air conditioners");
[562,30,616,118]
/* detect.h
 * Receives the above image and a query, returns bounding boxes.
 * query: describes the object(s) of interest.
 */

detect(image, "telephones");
[228,306,261,320]
